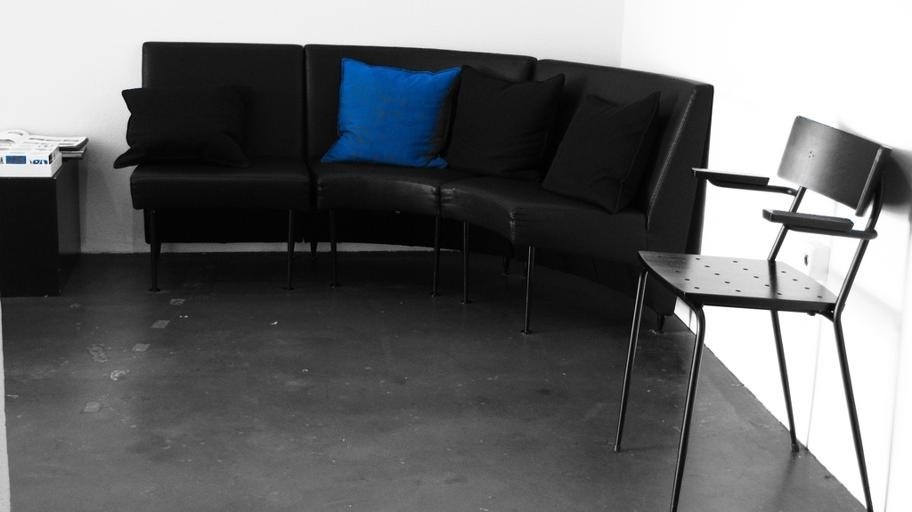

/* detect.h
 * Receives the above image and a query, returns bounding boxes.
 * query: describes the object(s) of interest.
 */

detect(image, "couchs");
[131,41,714,336]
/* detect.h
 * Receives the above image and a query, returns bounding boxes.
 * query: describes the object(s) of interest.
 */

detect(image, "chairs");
[614,112,891,512]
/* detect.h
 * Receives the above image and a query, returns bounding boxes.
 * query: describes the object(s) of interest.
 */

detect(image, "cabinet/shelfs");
[0,158,81,296]
